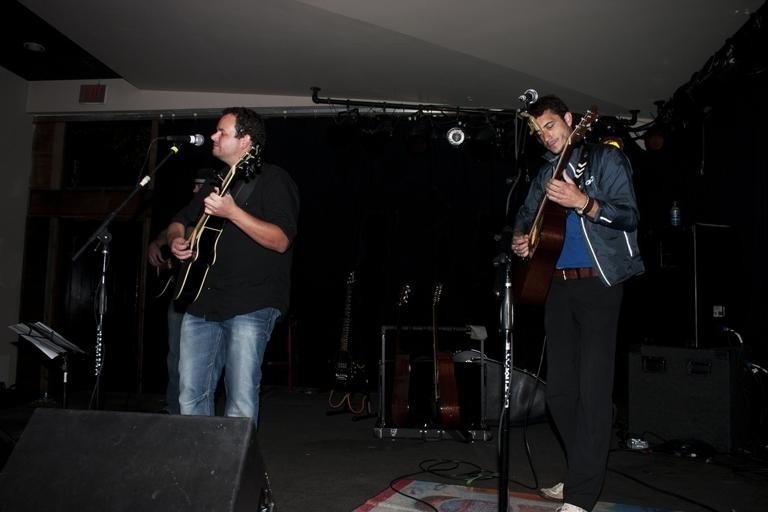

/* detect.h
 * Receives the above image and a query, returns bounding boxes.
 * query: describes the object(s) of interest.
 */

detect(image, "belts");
[551,267,602,282]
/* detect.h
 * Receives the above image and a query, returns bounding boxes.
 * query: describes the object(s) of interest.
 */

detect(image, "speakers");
[0,407,276,512]
[630,344,729,453]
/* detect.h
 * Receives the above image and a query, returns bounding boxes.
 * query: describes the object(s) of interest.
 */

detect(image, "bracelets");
[575,195,594,217]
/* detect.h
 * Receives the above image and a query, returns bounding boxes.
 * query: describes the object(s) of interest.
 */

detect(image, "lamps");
[306,85,628,154]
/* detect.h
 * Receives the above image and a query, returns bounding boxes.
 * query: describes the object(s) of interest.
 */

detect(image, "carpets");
[345,474,658,512]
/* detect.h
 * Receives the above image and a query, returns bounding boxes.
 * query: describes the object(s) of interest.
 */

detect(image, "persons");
[511,92,641,512]
[146,166,216,411]
[166,105,300,443]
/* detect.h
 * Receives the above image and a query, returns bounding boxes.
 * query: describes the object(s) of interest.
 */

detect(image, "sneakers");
[555,502,590,512]
[537,482,568,502]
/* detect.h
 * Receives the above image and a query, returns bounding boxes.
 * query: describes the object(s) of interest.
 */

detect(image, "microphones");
[158,134,205,146]
[519,88,538,104]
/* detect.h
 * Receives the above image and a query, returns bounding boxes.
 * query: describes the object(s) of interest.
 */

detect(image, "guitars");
[325,272,355,392]
[173,138,265,306]
[513,103,599,308]
[387,280,416,426]
[429,282,462,428]
